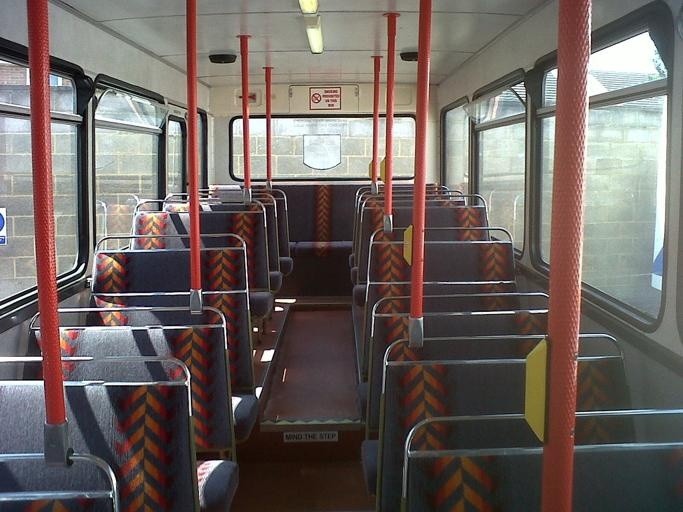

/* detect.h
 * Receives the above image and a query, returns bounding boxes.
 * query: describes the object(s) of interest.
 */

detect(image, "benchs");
[0,184,293,511]
[347,179,683,510]
[241,185,437,296]
